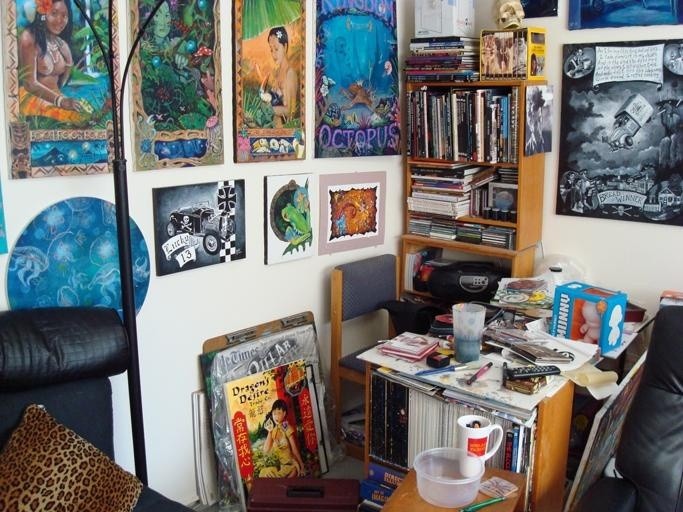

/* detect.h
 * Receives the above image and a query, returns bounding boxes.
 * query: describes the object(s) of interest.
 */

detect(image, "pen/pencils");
[460,497,506,512]
[467,362,493,385]
[412,364,468,376]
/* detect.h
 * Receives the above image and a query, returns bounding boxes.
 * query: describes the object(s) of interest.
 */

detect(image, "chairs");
[1,306,194,512]
[330,253,401,461]
[572,291,683,512]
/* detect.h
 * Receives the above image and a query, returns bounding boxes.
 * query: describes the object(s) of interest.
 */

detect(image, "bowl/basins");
[412,446,486,509]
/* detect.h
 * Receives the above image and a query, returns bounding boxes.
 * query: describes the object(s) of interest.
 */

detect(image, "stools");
[380,460,526,511]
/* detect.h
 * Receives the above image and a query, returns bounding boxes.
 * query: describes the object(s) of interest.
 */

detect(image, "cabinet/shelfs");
[399,77,550,305]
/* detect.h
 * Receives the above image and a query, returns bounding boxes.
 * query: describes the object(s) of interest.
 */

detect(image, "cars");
[165,201,238,257]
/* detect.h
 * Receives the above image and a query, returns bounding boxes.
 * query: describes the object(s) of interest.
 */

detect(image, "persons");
[525,89,546,154]
[17,0,92,126]
[254,27,297,128]
[530,52,538,76]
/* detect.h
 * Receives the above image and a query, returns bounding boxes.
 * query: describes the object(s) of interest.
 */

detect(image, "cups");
[458,414,502,477]
[452,303,486,362]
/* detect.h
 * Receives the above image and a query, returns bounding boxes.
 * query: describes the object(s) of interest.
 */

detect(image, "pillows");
[1,403,144,512]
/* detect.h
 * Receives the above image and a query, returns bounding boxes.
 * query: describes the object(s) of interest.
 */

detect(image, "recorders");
[426,261,509,302]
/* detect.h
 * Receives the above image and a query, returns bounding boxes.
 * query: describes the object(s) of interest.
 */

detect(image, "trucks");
[601,92,656,156]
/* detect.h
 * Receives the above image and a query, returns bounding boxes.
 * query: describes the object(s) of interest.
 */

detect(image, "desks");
[366,316,659,512]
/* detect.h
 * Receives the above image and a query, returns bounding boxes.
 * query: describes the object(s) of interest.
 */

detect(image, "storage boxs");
[247,478,362,512]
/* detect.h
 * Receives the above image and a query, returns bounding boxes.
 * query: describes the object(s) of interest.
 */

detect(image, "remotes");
[505,365,561,380]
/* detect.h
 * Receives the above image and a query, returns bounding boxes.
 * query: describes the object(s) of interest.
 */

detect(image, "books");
[481,327,575,366]
[223,360,324,512]
[368,370,535,495]
[491,276,554,308]
[379,331,441,363]
[404,24,532,291]
